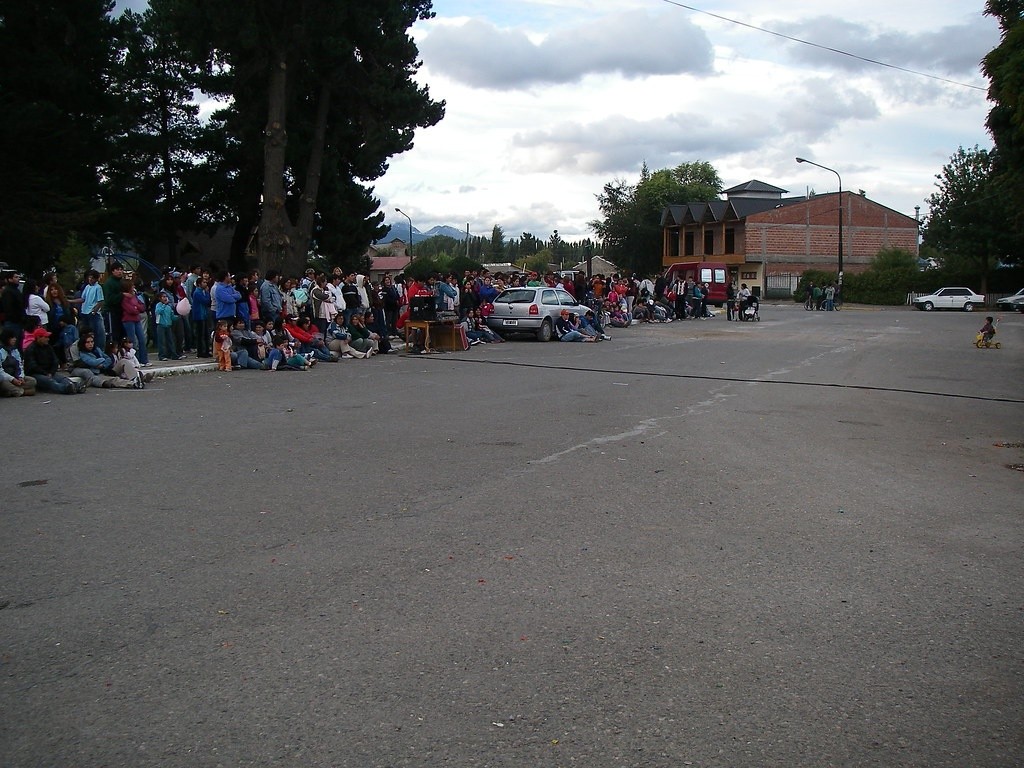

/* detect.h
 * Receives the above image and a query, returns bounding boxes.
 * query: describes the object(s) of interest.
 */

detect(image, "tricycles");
[973,331,1001,349]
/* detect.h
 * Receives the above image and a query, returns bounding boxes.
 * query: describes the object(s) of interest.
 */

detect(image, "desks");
[405,319,457,353]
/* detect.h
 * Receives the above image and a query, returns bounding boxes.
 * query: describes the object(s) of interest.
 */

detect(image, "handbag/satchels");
[399,295,406,306]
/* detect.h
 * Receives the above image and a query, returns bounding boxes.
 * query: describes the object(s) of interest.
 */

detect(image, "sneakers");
[134,371,145,389]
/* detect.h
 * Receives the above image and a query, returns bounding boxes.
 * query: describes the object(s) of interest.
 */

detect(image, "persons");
[424,269,574,346]
[805,281,841,311]
[573,271,715,328]
[726,283,751,322]
[554,309,612,342]
[980,317,995,342]
[0,261,431,397]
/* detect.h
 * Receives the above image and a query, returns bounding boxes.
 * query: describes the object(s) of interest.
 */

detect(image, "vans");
[663,263,728,309]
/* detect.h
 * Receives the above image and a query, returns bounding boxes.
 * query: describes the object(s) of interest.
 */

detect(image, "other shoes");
[366,347,372,358]
[309,359,317,369]
[208,353,213,357]
[76,377,93,393]
[342,354,354,358]
[198,354,209,358]
[162,358,167,361]
[594,333,601,342]
[471,341,480,346]
[388,348,398,353]
[177,356,182,360]
[140,362,153,367]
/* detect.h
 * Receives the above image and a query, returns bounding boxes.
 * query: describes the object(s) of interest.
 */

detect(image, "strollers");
[744,295,760,322]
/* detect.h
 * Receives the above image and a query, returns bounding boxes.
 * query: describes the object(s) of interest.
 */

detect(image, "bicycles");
[804,296,818,311]
[732,296,739,322]
[821,293,843,311]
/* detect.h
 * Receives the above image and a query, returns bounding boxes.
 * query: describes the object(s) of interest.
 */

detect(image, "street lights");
[395,208,413,266]
[795,157,844,306]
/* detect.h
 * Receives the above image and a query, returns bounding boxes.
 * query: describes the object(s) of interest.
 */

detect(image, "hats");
[306,268,315,274]
[561,309,570,315]
[34,328,52,338]
[532,272,537,276]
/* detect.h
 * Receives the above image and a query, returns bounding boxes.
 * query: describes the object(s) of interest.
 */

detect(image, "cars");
[487,286,592,342]
[995,288,1024,312]
[1013,297,1024,313]
[913,287,986,312]
